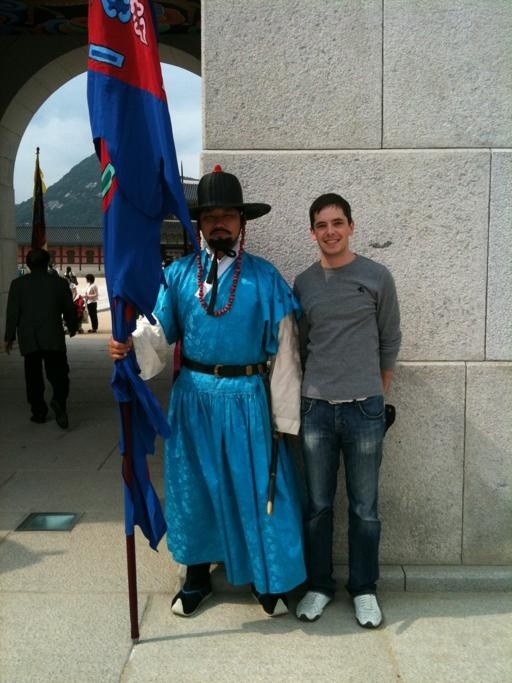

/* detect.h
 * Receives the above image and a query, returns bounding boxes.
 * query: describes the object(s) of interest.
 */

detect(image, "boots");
[170,562,213,617]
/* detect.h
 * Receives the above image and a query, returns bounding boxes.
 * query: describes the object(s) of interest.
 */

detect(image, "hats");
[187,165,271,221]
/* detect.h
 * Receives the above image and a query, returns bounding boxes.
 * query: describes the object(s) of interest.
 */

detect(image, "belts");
[182,356,267,378]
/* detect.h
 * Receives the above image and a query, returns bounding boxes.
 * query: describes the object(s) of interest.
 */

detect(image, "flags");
[30,153,47,250]
[88,0,199,554]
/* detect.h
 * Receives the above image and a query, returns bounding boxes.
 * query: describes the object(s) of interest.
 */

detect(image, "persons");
[64,274,86,335]
[106,166,304,617]
[47,263,58,275]
[291,191,402,628]
[3,247,80,430]
[84,273,100,332]
[63,265,75,281]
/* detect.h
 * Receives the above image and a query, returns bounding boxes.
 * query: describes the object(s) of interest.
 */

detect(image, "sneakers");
[250,582,290,617]
[296,590,334,623]
[354,584,382,628]
[30,415,48,424]
[50,399,69,430]
[88,328,97,332]
